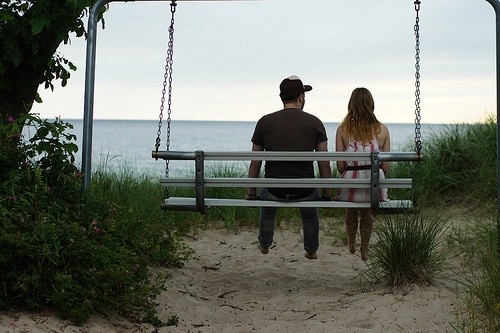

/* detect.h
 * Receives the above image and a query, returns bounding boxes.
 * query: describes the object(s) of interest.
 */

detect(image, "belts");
[269,190,308,199]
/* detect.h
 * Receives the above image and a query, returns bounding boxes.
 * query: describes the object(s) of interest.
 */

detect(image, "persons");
[334,88,390,260]
[246,75,331,259]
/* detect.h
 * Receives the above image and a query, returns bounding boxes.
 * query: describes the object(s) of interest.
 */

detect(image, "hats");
[278,75,312,95]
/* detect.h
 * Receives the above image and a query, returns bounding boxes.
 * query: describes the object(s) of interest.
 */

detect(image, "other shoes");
[257,242,269,253]
[349,248,356,253]
[361,253,368,260]
[305,252,317,259]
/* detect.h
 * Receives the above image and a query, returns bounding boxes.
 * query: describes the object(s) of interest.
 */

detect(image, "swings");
[151,1,424,214]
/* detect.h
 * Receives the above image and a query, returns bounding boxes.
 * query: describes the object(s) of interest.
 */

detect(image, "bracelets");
[247,193,256,196]
